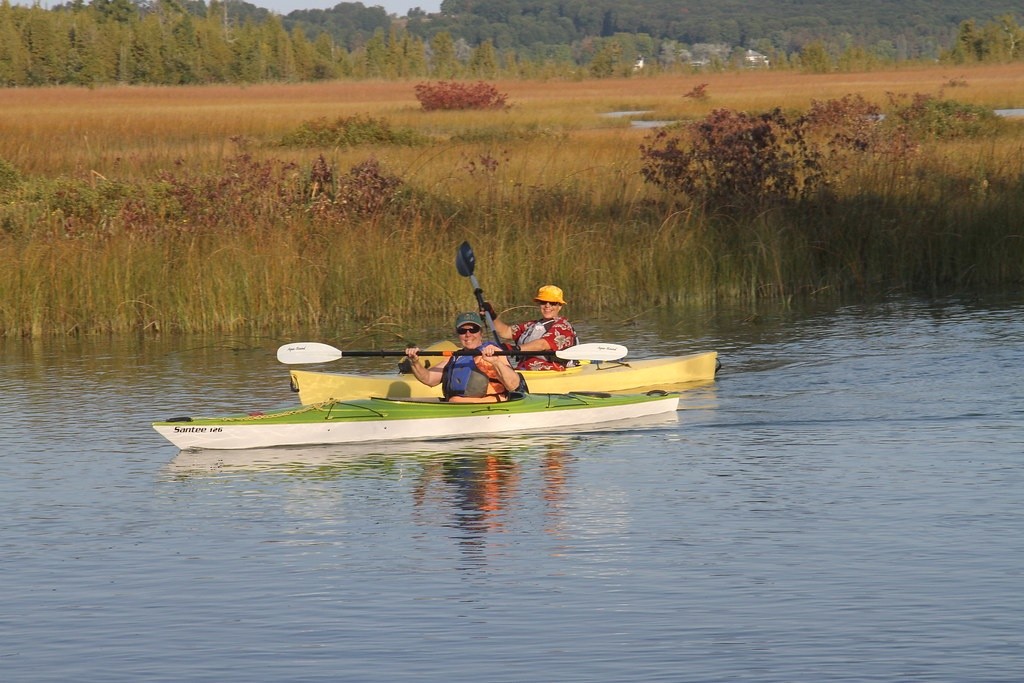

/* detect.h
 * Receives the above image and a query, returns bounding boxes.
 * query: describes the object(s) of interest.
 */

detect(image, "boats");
[152,393,681,449]
[289,351,721,405]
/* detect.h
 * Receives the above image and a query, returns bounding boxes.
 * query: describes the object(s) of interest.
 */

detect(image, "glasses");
[538,300,558,306]
[457,327,479,334]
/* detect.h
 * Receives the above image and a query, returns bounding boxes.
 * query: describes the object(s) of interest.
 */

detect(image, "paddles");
[455,241,529,394]
[276,342,628,364]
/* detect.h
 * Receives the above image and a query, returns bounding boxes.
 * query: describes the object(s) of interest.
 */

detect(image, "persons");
[479,284,575,372]
[404,312,520,402]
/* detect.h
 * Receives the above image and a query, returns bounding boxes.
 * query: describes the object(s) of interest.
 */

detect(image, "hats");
[455,312,483,329]
[533,285,567,307]
